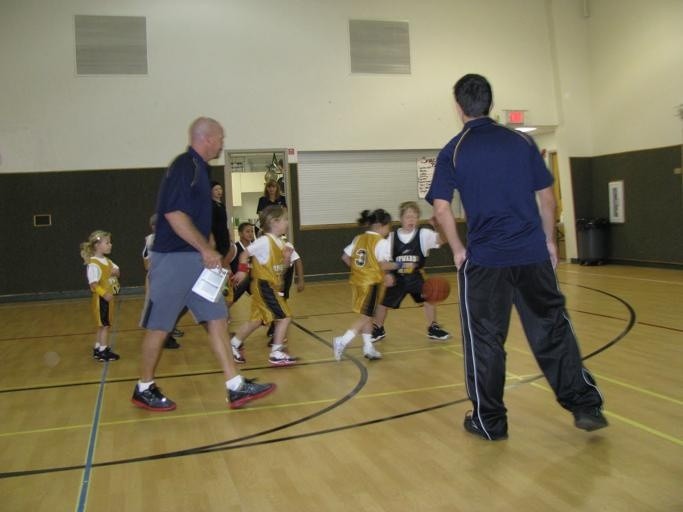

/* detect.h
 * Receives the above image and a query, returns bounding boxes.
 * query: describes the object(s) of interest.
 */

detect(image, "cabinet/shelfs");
[229,152,285,207]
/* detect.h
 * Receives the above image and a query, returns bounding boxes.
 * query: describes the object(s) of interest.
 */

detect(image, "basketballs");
[423,276,448,302]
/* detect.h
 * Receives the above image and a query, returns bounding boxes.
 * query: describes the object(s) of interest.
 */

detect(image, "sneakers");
[131,383,177,411]
[574,410,608,431]
[464,410,481,434]
[227,377,276,409]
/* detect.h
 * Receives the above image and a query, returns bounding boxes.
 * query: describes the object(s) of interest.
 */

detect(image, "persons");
[227,205,307,368]
[143,212,184,350]
[221,223,279,341]
[332,208,401,366]
[423,73,609,441]
[129,116,279,413]
[364,199,452,346]
[205,182,230,268]
[79,230,121,364]
[254,180,289,233]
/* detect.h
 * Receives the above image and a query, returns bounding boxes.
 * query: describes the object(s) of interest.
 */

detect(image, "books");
[190,262,228,304]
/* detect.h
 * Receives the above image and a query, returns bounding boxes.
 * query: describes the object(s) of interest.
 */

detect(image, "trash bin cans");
[576,216,606,266]
